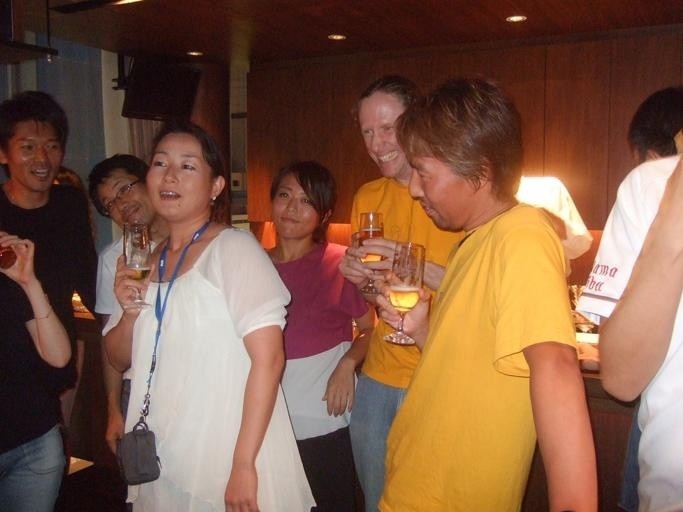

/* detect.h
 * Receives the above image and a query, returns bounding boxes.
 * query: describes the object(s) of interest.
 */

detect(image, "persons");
[0,89,99,432]
[376,74,601,511]
[575,148,683,512]
[88,152,171,460]
[101,110,318,511]
[263,157,378,511]
[627,82,683,164]
[0,230,72,512]
[337,75,469,512]
[52,165,96,244]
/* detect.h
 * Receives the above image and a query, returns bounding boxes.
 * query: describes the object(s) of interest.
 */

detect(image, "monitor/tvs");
[120,47,202,124]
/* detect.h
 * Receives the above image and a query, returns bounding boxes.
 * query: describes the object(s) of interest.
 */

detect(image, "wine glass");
[382,241,425,344]
[358,212,384,297]
[122,221,154,313]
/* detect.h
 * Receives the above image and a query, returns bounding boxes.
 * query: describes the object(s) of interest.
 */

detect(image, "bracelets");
[34,307,53,320]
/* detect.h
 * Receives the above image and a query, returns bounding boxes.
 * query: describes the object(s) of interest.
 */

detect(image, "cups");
[0,234,17,270]
[568,284,594,333]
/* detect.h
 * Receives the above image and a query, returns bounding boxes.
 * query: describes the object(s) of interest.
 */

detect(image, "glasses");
[102,180,140,216]
